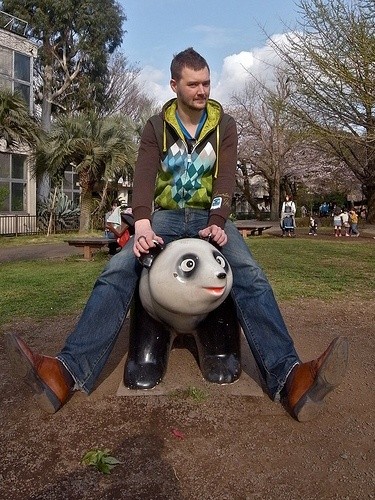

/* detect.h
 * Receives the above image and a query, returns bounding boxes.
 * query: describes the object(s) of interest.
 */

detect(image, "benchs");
[235,225,273,237]
[63,238,117,262]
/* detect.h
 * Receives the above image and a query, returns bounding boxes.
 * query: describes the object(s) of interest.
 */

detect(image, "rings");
[137,235,146,242]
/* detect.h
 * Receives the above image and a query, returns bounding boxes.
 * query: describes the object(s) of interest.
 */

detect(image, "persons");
[280,194,297,237]
[308,216,318,236]
[104,200,130,254]
[333,207,360,238]
[7,48,348,422]
[301,206,306,218]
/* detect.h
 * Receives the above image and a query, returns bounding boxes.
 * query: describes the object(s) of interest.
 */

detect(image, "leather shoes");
[6,332,71,414]
[284,336,349,423]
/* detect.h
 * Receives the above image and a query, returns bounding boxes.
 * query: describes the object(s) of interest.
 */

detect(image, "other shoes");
[314,233,317,236]
[309,233,312,235]
[335,233,360,238]
